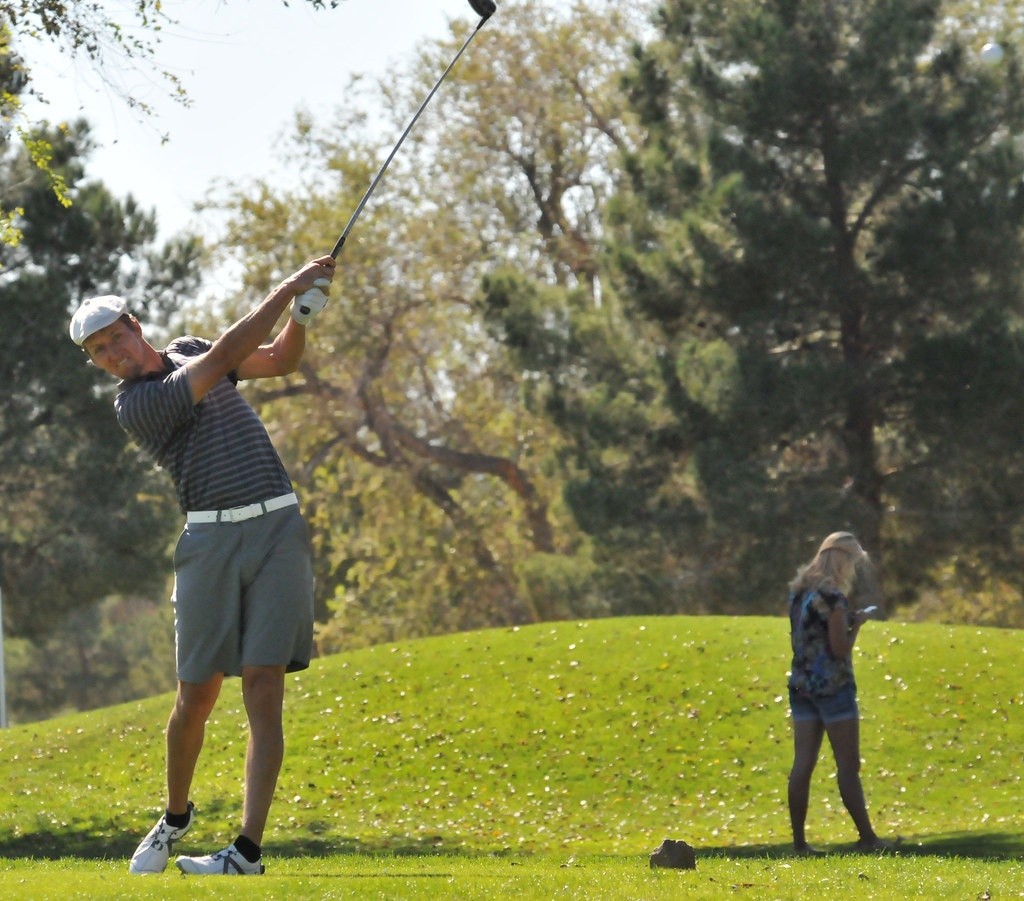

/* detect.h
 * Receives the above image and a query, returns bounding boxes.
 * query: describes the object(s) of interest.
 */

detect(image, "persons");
[69,254,334,873]
[786,530,893,859]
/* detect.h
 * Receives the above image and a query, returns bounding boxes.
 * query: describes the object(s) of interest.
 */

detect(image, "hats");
[70,294,126,347]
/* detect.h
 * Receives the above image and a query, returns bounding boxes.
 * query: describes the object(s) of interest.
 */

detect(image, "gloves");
[290,276,339,333]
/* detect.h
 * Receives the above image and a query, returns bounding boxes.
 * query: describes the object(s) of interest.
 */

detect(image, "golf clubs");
[298,0,498,316]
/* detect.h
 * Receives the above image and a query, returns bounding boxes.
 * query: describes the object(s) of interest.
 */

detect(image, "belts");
[186,492,299,525]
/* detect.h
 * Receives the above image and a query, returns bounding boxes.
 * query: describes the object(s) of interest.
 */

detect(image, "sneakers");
[175,842,265,878]
[128,799,194,875]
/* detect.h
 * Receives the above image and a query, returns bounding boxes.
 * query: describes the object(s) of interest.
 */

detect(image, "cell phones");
[866,605,878,613]
